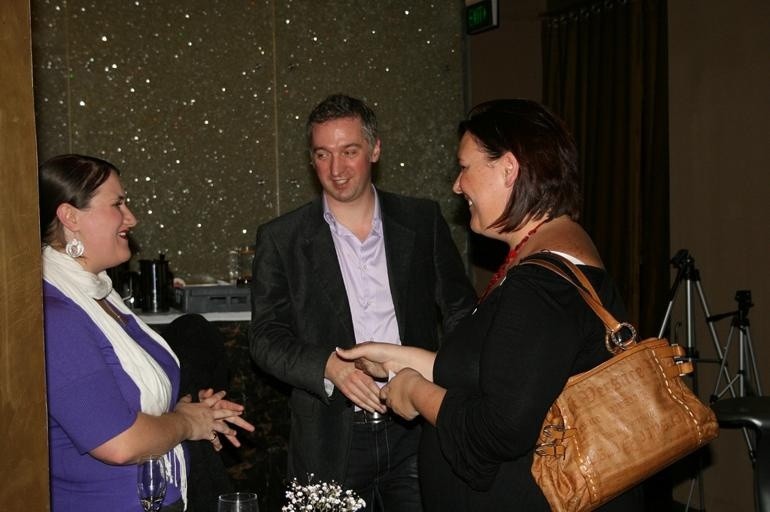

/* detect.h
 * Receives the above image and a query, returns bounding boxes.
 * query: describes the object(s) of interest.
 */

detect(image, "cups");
[138,258,170,313]
[218,492,259,512]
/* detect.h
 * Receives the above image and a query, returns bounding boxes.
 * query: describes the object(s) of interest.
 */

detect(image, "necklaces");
[471,209,568,313]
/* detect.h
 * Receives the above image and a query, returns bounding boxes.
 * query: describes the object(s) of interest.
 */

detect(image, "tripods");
[683,288,770,512]
[657,248,755,511]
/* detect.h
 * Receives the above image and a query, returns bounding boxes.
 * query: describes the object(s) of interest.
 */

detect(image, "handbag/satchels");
[517,251,719,512]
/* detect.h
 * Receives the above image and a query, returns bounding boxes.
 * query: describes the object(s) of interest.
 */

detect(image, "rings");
[210,432,217,442]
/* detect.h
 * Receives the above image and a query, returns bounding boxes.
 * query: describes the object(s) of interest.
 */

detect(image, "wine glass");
[136,454,166,511]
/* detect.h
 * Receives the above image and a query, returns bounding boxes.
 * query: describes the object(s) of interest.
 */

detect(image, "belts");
[354,408,397,425]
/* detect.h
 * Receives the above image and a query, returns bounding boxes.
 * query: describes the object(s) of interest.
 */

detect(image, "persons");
[248,95,481,511]
[38,154,255,512]
[334,101,629,511]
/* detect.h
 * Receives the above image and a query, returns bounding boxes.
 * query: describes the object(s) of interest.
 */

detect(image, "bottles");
[229,244,255,288]
